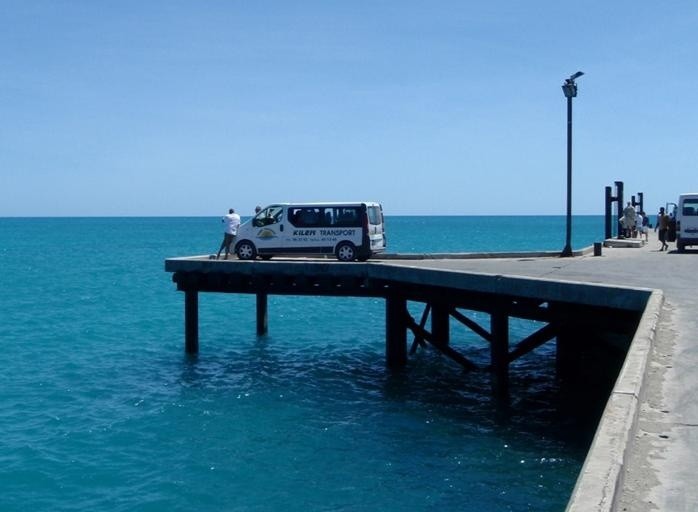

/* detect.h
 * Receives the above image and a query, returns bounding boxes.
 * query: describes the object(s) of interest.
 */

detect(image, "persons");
[654,207,670,251]
[216,208,240,260]
[255,205,265,218]
[619,201,648,238]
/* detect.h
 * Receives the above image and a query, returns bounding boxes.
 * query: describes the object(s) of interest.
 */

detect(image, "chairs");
[683,207,695,215]
[290,207,360,227]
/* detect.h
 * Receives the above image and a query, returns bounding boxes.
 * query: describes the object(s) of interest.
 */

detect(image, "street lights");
[558,70,584,258]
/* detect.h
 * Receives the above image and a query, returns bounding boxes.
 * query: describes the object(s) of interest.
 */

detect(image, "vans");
[665,194,697,250]
[228,201,385,262]
